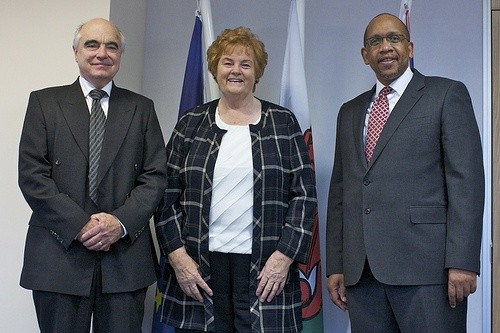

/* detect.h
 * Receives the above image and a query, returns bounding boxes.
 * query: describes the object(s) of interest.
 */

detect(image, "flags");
[178,0,220,118]
[399,0,414,68]
[278,0,325,333]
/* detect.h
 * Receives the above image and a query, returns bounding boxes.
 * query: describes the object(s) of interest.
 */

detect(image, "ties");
[364,85,394,165]
[88,89,107,208]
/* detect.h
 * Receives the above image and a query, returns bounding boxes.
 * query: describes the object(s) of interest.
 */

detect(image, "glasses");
[365,34,410,48]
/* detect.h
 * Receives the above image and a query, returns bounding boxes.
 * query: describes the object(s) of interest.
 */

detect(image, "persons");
[326,13,485,333]
[18,18,169,333]
[153,25,317,333]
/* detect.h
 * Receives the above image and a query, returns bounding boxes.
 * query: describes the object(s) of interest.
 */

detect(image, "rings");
[99,241,103,246]
